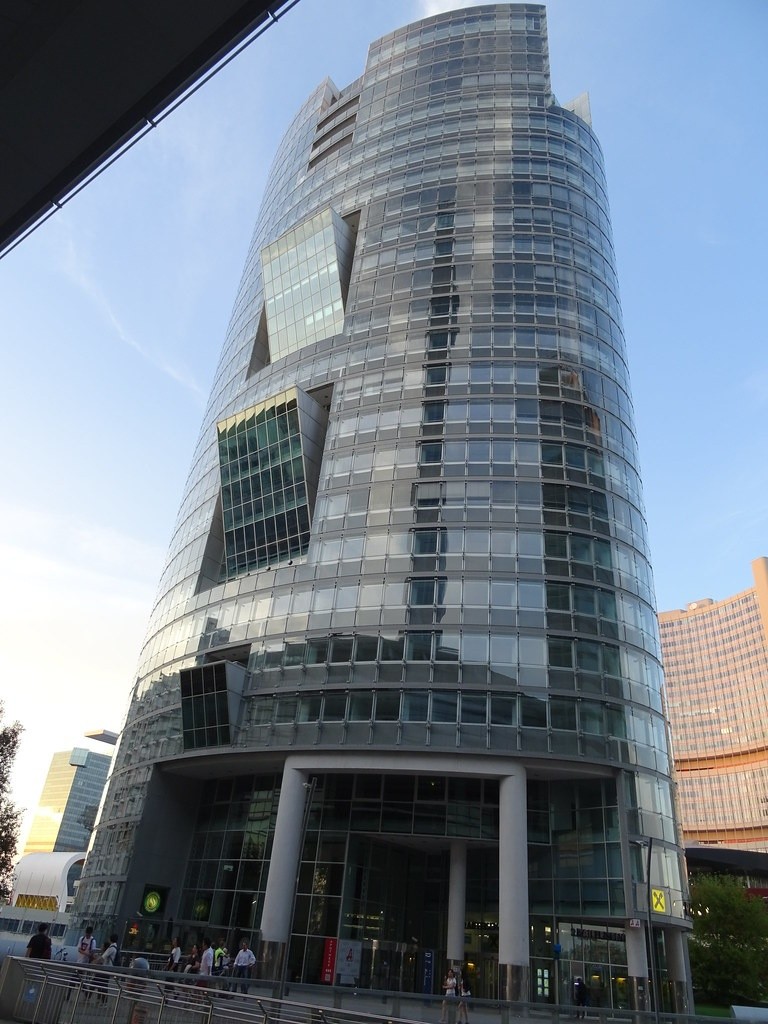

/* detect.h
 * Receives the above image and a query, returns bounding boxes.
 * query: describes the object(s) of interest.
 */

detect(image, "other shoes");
[457,1021,462,1024]
[98,1004,107,1009]
[574,1016,579,1019]
[82,998,90,1003]
[581,1016,584,1019]
[438,1019,446,1023]
[173,992,179,1000]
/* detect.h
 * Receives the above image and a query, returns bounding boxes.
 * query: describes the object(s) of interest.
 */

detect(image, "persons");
[576,978,587,1019]
[24,924,53,962]
[441,970,471,1024]
[64,927,257,1008]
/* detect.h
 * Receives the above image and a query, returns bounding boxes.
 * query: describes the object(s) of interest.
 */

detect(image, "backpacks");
[109,946,121,966]
